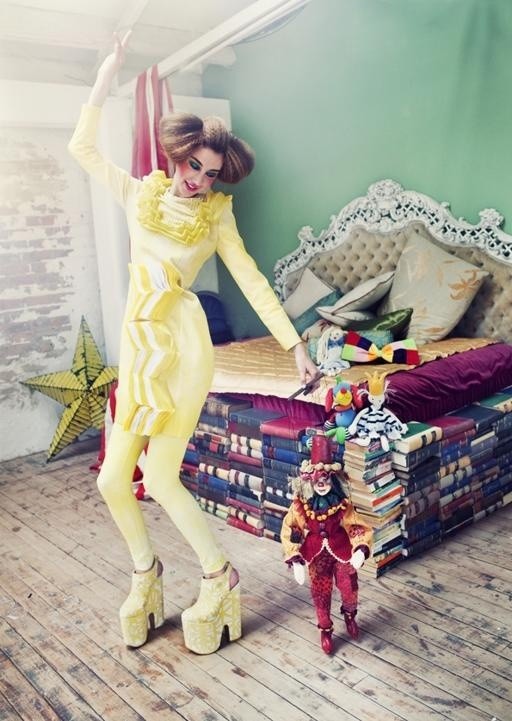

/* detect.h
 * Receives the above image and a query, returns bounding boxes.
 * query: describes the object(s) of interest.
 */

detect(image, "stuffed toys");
[322,381,362,436]
[314,324,351,374]
[347,372,408,451]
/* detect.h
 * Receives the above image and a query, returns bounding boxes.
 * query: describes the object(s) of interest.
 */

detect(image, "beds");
[180,181,512,579]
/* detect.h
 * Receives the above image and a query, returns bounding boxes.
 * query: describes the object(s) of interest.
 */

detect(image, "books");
[332,388,512,579]
[181,398,322,543]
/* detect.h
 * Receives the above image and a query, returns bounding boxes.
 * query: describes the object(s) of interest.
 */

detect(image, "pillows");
[282,232,489,365]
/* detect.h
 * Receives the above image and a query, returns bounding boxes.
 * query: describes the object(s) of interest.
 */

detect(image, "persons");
[279,476,374,654]
[65,26,320,653]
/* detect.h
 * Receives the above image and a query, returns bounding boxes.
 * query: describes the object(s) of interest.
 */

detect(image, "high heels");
[120,558,164,647]
[181,564,241,655]
